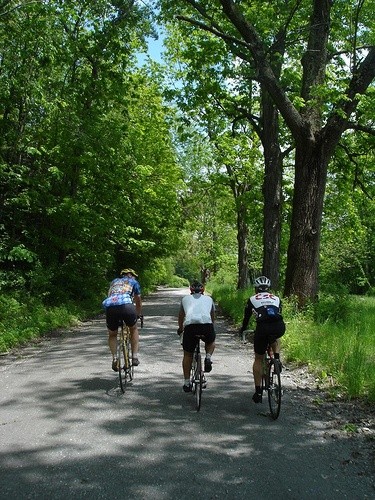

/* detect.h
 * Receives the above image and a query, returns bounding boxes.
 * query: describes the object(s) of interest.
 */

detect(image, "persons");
[239,276,286,403]
[177,279,217,393]
[102,268,142,371]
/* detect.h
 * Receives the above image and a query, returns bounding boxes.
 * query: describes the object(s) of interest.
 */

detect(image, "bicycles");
[177,329,211,412]
[116,315,144,393]
[242,329,282,420]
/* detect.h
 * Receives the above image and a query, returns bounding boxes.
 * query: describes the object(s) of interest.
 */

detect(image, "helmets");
[190,281,205,294]
[120,268,139,277]
[252,276,272,288]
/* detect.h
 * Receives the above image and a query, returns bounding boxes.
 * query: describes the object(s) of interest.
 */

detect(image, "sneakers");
[132,358,140,366]
[205,358,212,372]
[112,361,119,371]
[275,359,282,374]
[183,384,191,393]
[252,392,262,404]
